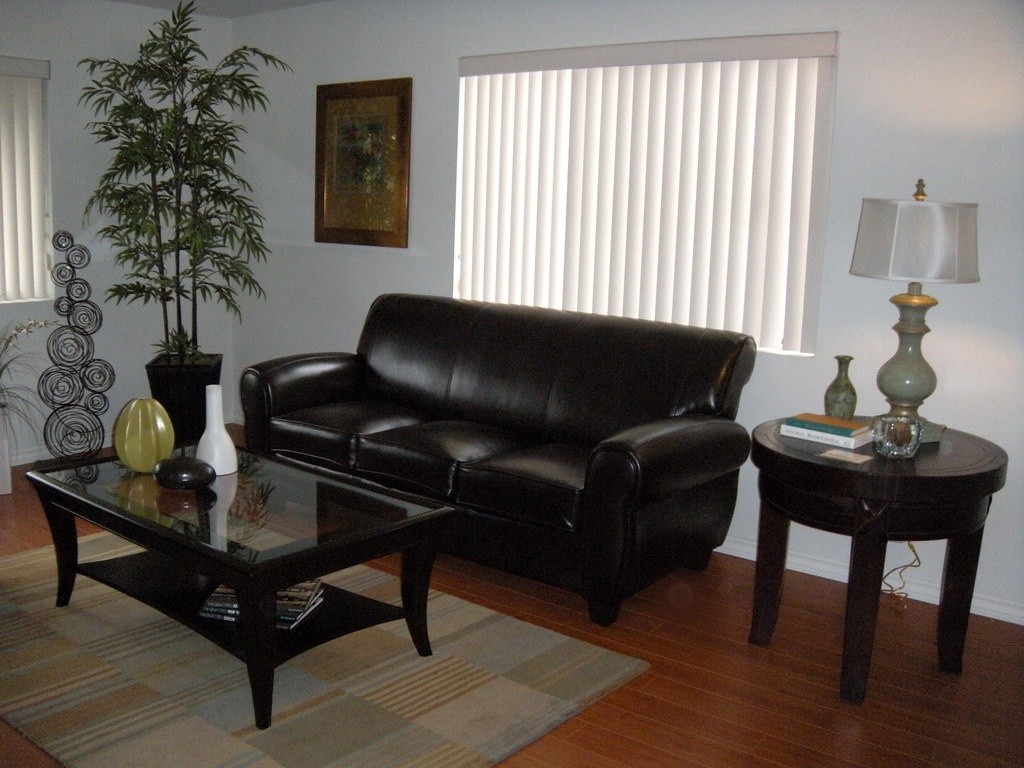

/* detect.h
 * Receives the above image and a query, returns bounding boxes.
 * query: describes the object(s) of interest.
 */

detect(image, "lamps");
[844,176,985,460]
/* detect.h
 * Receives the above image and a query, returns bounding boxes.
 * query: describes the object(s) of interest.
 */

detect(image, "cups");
[870,415,924,459]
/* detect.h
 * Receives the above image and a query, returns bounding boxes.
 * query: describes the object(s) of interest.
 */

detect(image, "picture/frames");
[313,75,412,250]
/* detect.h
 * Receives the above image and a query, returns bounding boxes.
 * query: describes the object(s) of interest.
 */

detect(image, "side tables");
[741,411,1007,704]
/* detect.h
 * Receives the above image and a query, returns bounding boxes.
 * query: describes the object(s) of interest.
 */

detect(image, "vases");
[111,396,178,476]
[823,352,857,417]
[195,380,240,474]
[208,472,237,553]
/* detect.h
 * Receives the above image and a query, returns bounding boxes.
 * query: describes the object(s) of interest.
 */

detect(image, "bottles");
[113,398,175,474]
[823,355,858,420]
[196,384,239,476]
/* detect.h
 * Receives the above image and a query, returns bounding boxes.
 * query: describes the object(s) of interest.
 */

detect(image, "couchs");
[236,290,756,632]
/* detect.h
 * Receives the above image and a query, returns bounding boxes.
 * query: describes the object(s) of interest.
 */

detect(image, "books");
[199,578,324,630]
[780,413,874,449]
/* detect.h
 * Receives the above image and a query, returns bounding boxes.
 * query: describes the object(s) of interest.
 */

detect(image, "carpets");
[0,527,654,768]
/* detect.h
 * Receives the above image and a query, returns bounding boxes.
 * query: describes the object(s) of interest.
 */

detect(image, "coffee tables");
[21,444,456,732]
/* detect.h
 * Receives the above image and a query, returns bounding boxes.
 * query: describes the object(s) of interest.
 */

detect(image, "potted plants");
[75,0,296,445]
